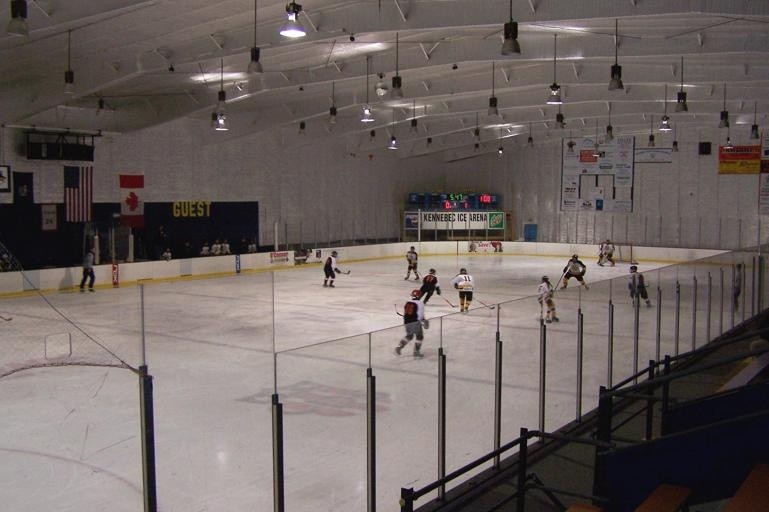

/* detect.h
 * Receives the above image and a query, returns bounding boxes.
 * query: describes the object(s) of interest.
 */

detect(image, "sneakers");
[546,316,559,323]
[395,346,402,355]
[413,351,424,357]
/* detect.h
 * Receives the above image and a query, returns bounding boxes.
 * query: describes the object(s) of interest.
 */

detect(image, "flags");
[118,170,146,229]
[64,167,94,223]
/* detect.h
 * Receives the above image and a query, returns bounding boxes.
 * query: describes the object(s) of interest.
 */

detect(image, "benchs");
[565,350,768,512]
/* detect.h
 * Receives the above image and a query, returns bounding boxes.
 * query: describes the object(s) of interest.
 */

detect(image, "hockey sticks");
[335,271,350,274]
[439,294,460,309]
[395,304,426,326]
[0,315,13,322]
[473,298,495,310]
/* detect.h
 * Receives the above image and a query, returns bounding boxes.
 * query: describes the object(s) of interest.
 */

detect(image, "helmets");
[411,268,466,296]
[541,255,637,281]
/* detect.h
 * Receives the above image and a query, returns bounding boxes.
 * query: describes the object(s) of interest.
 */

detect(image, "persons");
[79,245,96,293]
[323,250,341,288]
[162,236,256,259]
[394,239,651,357]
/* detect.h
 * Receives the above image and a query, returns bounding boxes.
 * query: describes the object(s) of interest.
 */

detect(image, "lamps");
[607,17,626,91]
[242,1,266,77]
[95,98,106,116]
[4,1,31,39]
[500,0,522,57]
[277,1,308,39]
[294,29,759,154]
[208,57,233,132]
[58,28,79,96]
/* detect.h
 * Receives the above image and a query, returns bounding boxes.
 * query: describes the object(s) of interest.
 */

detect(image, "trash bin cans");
[524,224,537,242]
